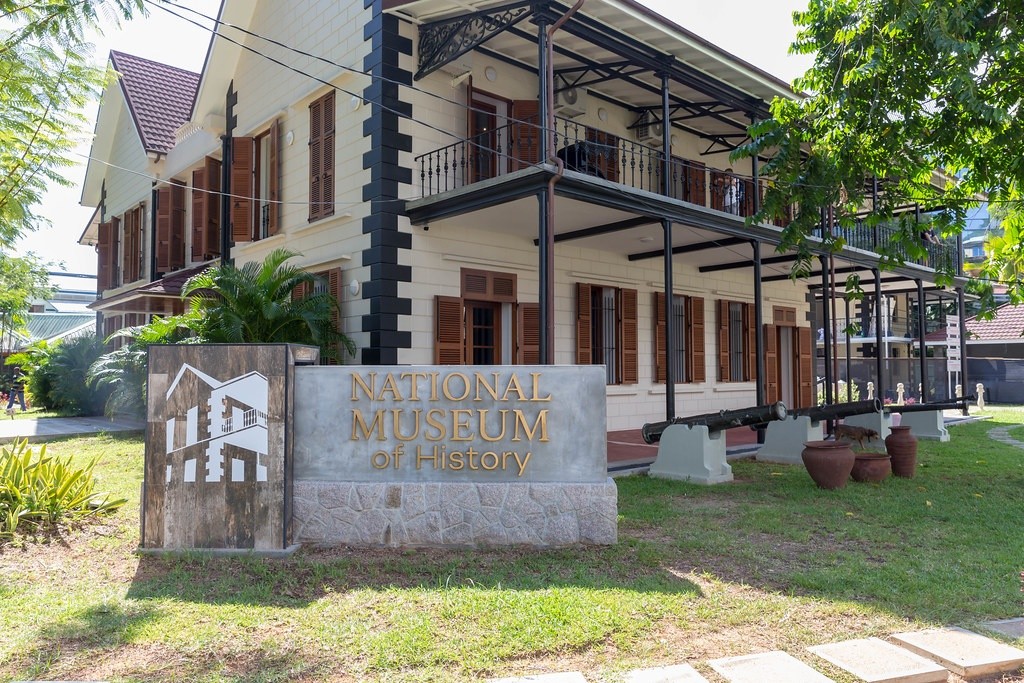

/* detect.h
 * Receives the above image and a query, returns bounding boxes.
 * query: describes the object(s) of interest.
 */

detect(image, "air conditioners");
[553,76,587,121]
[639,111,671,147]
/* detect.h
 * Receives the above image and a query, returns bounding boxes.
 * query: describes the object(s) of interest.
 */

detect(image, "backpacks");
[739,179,746,202]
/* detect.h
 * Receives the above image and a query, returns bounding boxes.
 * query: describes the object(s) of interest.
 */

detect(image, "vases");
[850,453,891,482]
[801,440,855,489]
[884,426,918,477]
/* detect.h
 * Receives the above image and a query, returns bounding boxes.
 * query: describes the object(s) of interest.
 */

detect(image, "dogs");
[824,425,879,450]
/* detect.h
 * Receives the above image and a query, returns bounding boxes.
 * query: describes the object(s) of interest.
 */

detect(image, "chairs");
[556,142,606,179]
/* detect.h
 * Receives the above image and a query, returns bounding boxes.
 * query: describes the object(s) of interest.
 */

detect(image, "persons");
[6,367,27,411]
[920,219,943,267]
[715,168,740,216]
